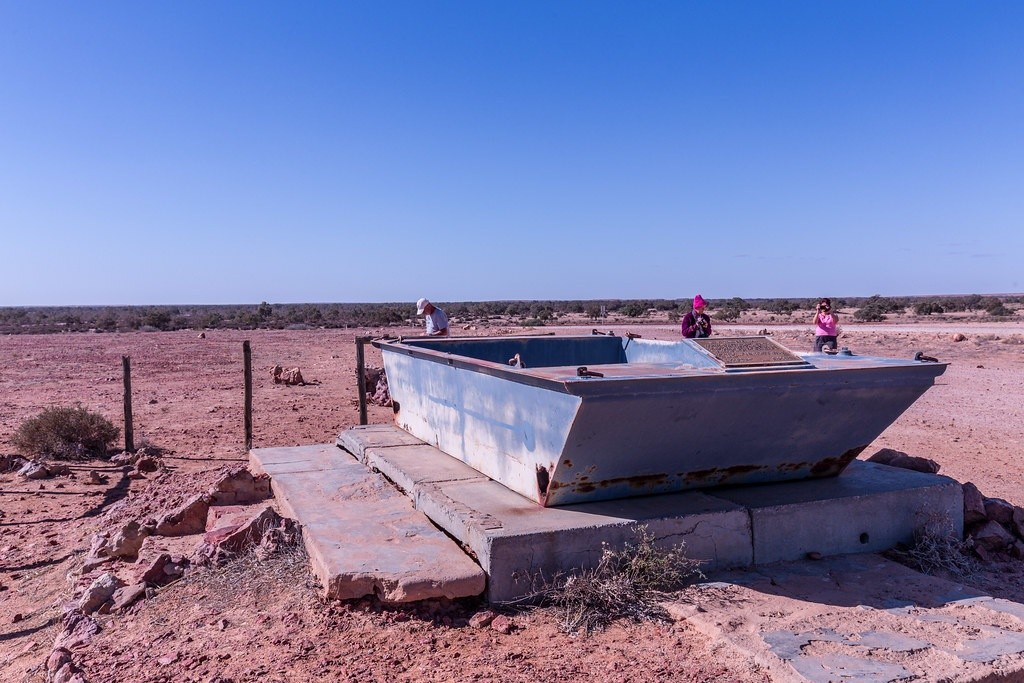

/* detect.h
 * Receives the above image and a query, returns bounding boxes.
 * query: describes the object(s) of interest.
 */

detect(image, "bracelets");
[428,333,432,336]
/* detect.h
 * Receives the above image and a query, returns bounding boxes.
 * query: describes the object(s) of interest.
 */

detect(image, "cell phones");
[820,306,826,309]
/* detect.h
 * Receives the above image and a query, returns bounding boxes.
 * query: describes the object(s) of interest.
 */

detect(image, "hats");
[417,298,429,314]
[694,295,706,308]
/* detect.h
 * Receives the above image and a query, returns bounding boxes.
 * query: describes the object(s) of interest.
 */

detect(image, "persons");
[682,295,712,338]
[417,298,450,336]
[813,298,838,351]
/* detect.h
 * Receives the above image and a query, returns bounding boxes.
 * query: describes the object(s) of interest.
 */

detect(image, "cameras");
[699,319,705,326]
